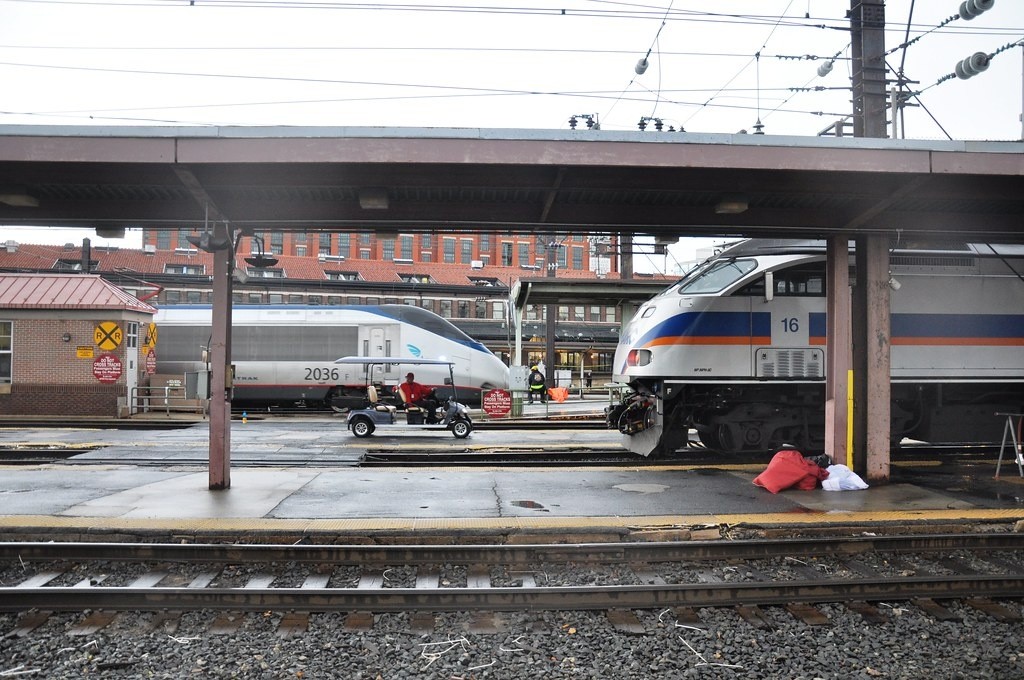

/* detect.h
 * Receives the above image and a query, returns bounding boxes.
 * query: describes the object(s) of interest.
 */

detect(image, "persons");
[587,373,592,389]
[392,373,442,424]
[528,366,546,403]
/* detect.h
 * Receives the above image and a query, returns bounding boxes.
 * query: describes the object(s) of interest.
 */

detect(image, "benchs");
[367,385,397,412]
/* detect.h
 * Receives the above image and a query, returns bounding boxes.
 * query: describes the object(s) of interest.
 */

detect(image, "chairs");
[398,385,425,413]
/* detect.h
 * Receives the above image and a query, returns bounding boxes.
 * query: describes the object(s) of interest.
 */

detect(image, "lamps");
[62,332,71,341]
[244,235,279,268]
[96,225,125,238]
[654,234,679,245]
[888,275,900,291]
[359,187,390,210]
[714,201,749,214]
[188,201,231,252]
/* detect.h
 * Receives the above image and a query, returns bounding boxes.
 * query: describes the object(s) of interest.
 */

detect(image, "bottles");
[242,411,247,423]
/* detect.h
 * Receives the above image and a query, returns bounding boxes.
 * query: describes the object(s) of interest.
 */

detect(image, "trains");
[602,238,1024,458]
[147,304,512,405]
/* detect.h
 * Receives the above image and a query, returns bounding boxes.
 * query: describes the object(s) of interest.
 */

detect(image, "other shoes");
[528,400,533,403]
[427,414,442,422]
[540,400,546,403]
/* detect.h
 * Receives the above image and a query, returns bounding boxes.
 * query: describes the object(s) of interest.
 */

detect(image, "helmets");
[530,365,539,371]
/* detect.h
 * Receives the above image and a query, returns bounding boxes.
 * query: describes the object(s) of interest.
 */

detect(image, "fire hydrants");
[241,412,248,424]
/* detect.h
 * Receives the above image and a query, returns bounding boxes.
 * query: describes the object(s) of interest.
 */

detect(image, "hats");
[405,373,414,379]
